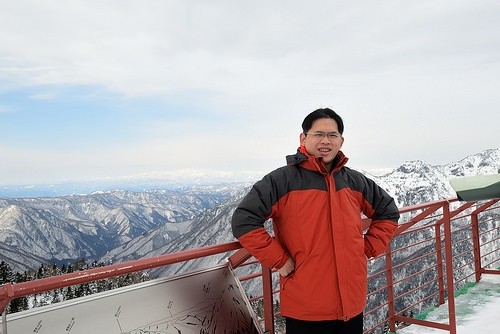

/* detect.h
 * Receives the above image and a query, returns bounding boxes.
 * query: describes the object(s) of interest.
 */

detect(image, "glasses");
[305,132,341,140]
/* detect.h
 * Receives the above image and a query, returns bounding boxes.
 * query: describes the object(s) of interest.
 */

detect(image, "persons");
[231,108,400,334]
